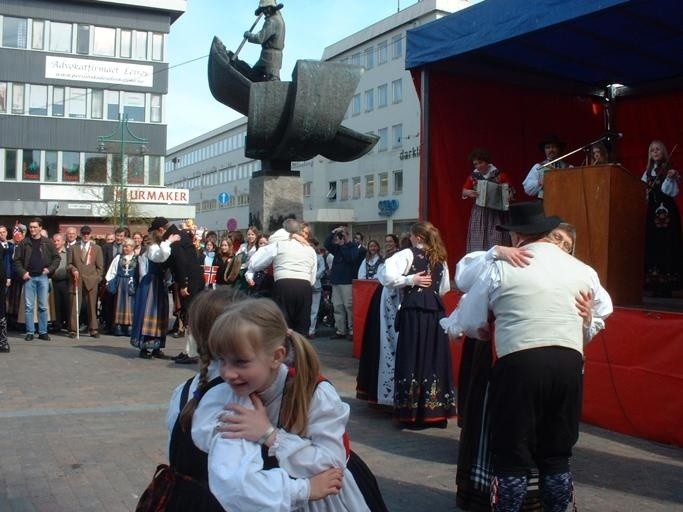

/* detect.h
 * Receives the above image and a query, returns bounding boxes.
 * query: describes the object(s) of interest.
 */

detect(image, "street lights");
[96,110,153,232]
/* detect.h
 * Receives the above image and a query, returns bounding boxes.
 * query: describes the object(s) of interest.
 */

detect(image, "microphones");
[608,132,624,142]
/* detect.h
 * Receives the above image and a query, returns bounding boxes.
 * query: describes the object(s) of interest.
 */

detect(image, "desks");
[542,164,647,306]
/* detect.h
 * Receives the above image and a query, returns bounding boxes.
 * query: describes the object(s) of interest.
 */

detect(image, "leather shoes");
[171,351,199,364]
[91,331,100,338]
[70,332,75,338]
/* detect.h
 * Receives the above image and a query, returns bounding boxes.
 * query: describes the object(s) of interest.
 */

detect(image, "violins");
[654,165,682,181]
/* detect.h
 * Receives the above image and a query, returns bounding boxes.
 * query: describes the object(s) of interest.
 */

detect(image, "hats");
[538,132,568,151]
[496,202,560,235]
[148,217,168,232]
[81,226,91,232]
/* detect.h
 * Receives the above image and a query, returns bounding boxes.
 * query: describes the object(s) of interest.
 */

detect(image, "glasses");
[508,230,514,235]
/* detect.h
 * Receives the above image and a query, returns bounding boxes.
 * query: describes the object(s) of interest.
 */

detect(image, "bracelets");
[257,426,275,444]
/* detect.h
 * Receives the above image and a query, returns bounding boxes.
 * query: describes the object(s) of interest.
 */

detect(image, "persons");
[0,217,206,366]
[460,149,515,255]
[377,222,458,429]
[589,145,609,165]
[209,296,388,512]
[454,224,607,511]
[243,0,285,83]
[198,219,412,340]
[132,285,344,512]
[641,140,683,298]
[355,232,418,410]
[444,202,612,511]
[521,135,570,203]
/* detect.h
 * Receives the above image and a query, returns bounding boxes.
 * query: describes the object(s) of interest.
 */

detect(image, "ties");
[82,246,87,259]
[5,244,8,250]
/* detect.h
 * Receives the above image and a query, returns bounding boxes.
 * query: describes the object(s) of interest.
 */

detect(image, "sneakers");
[330,333,347,340]
[26,334,33,341]
[152,350,164,358]
[139,350,152,359]
[39,334,52,340]
[347,335,353,341]
